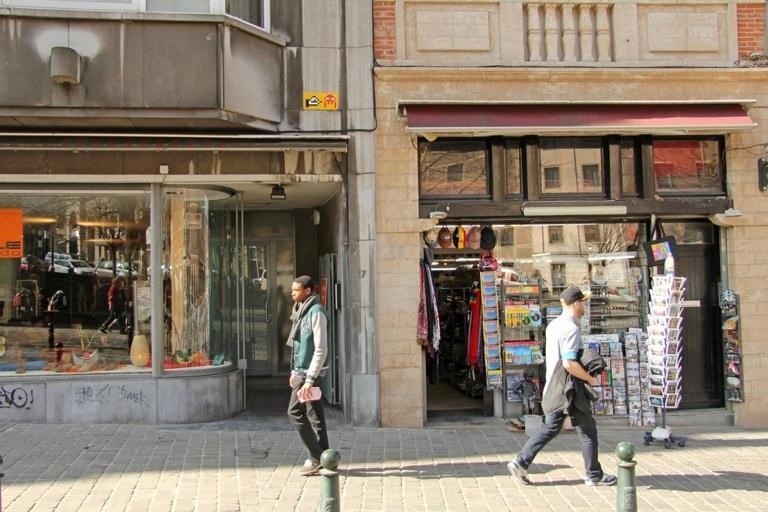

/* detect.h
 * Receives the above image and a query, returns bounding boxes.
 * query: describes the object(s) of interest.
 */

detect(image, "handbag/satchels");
[643,218,678,267]
[479,248,497,271]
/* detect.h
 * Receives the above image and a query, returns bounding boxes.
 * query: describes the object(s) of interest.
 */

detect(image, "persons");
[507,287,615,488]
[276,284,289,362]
[107,276,127,333]
[99,278,124,334]
[287,275,331,476]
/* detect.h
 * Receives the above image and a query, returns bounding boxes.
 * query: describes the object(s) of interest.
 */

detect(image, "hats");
[453,226,467,249]
[468,227,481,250]
[559,286,589,304]
[481,227,495,250]
[424,228,438,247]
[438,228,451,248]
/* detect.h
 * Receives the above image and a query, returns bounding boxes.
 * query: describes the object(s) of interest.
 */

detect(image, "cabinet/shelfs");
[434,285,485,400]
[542,280,641,344]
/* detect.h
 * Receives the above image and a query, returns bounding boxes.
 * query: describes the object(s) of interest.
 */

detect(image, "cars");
[585,283,640,314]
[16,249,271,293]
[494,265,526,288]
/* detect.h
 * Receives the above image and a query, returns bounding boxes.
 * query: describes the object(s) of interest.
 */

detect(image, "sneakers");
[507,461,533,485]
[300,461,322,476]
[585,474,617,486]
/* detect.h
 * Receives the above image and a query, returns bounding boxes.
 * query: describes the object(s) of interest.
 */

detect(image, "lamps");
[270,185,286,200]
[49,47,81,87]
[520,200,628,217]
